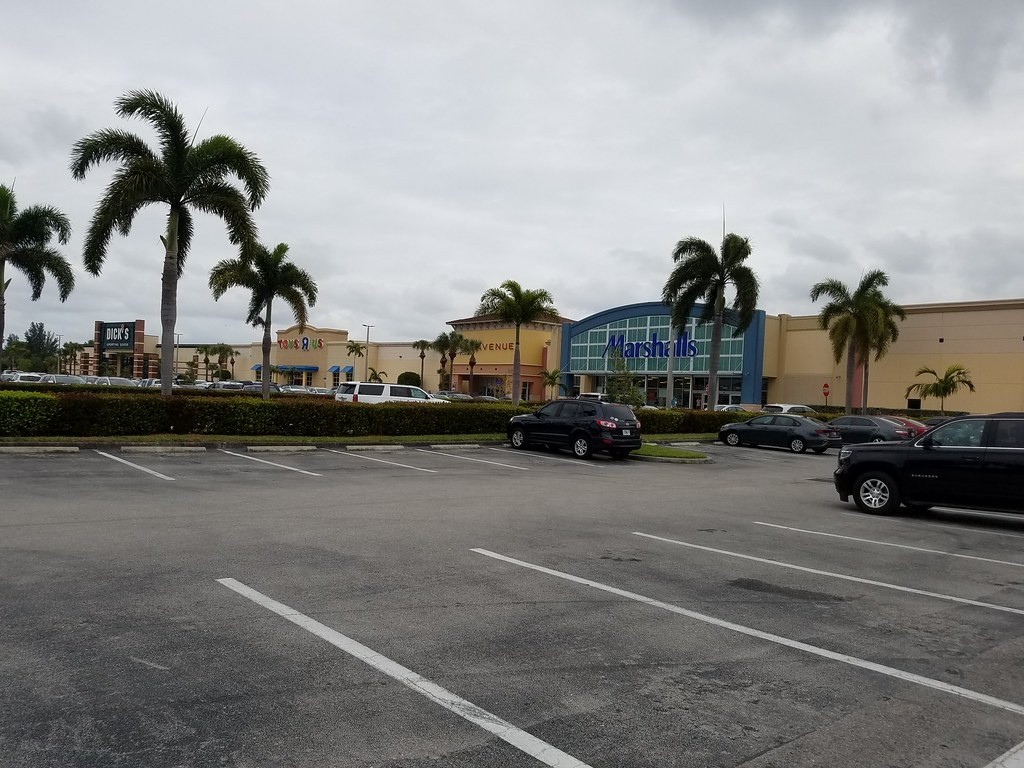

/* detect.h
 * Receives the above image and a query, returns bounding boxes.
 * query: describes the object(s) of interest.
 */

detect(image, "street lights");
[56,334,64,374]
[230,358,235,380]
[362,324,375,382]
[418,349,426,390]
[203,355,209,381]
[173,332,184,373]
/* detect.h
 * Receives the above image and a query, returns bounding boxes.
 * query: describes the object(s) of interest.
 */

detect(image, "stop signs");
[822,383,830,396]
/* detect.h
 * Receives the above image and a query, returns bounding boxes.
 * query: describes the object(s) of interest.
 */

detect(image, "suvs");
[759,403,818,414]
[575,392,660,411]
[334,380,452,404]
[832,410,1024,518]
[506,397,642,461]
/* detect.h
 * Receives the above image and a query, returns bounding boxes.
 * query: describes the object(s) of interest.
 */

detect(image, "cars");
[0,368,525,402]
[824,414,986,449]
[703,402,746,412]
[717,413,844,454]
